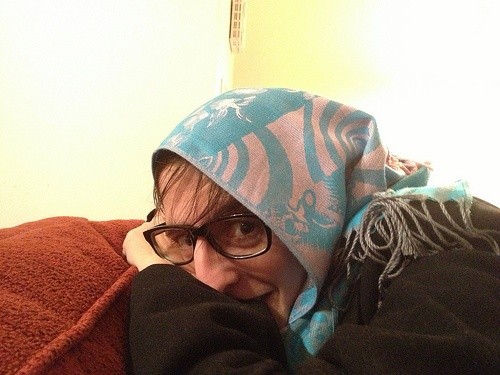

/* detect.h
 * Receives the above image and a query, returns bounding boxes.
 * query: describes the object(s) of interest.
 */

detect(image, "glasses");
[143,212,272,266]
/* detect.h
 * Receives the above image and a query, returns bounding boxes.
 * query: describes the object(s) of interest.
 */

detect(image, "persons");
[121,88,500,375]
